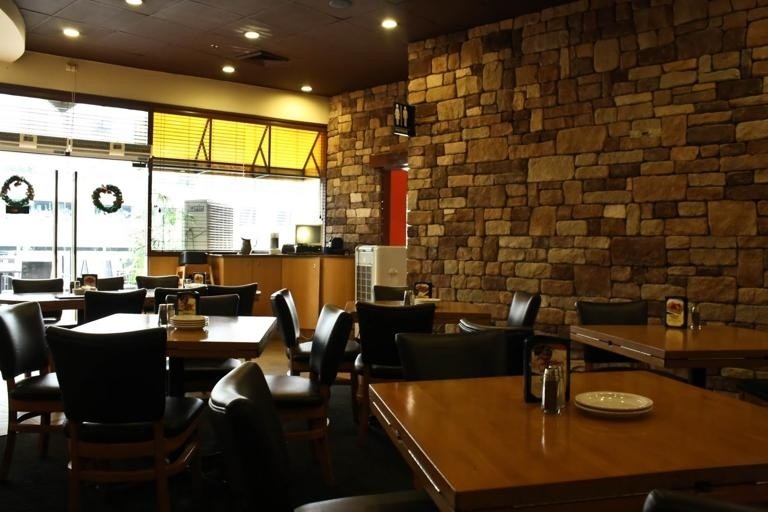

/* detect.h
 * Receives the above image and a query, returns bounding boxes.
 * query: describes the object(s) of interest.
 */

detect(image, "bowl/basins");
[72,289,85,295]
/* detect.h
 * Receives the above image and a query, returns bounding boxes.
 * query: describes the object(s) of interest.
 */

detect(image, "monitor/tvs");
[295,225,322,247]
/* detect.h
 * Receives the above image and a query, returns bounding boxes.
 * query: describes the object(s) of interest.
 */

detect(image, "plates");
[573,391,654,412]
[575,415,651,439]
[169,314,209,328]
[170,326,208,340]
[573,398,656,419]
[184,283,208,290]
[415,297,441,306]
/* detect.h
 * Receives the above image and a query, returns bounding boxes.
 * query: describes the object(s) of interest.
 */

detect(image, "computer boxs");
[296,246,322,253]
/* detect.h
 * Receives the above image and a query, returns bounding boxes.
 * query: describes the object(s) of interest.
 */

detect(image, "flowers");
[0,175,36,206]
[93,183,123,214]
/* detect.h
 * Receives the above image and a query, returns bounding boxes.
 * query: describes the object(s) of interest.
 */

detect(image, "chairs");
[723,378,767,405]
[353,261,542,434]
[293,489,439,512]
[0,261,360,512]
[643,489,763,510]
[574,299,648,373]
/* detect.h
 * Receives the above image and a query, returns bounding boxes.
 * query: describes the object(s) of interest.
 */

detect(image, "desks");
[366,369,768,512]
[570,325,767,374]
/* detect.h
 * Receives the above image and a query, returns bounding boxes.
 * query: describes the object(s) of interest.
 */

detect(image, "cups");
[270,233,279,249]
[74,281,80,289]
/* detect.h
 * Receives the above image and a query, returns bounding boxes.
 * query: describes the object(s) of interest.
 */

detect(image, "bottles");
[542,368,562,416]
[241,238,252,256]
[328,236,332,248]
[403,290,410,307]
[165,303,175,327]
[157,304,168,328]
[548,365,567,410]
[690,306,703,334]
[540,414,562,460]
[409,289,415,308]
